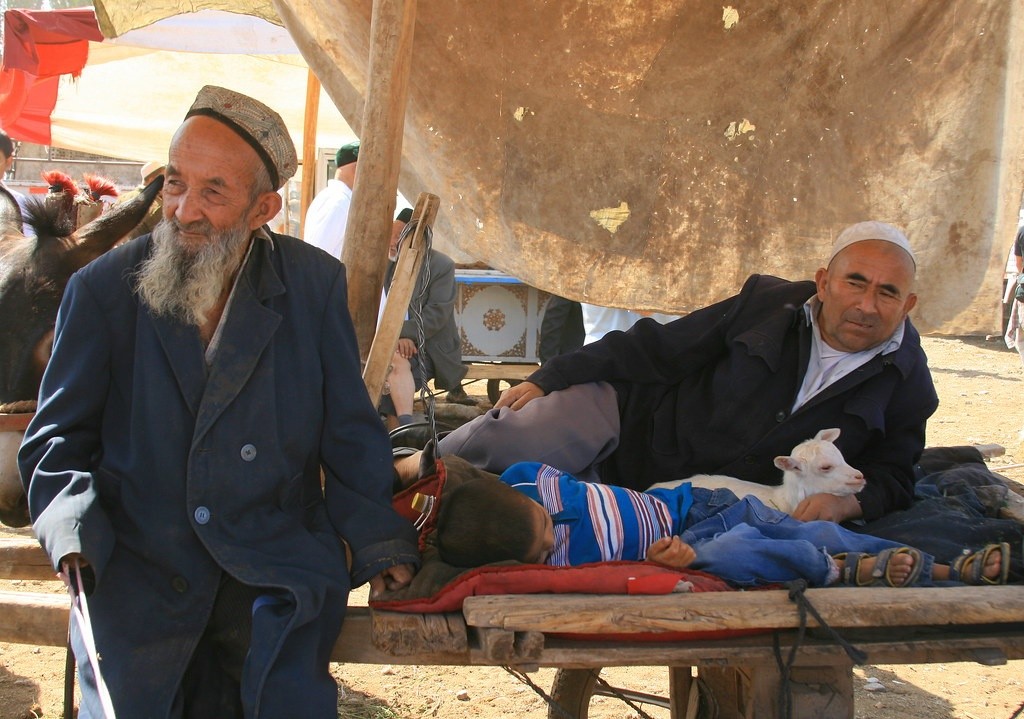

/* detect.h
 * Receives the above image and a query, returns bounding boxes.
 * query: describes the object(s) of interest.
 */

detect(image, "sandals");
[949,542,1010,585]
[833,546,924,587]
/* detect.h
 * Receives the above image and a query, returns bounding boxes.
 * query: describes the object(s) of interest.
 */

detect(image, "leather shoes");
[390,446,419,496]
[389,421,458,450]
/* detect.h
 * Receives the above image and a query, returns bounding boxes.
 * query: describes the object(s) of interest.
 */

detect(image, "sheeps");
[643,427,866,516]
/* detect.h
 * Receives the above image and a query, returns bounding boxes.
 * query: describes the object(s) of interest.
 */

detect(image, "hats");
[397,208,413,224]
[185,84,299,193]
[336,142,360,167]
[826,223,917,276]
[140,162,164,179]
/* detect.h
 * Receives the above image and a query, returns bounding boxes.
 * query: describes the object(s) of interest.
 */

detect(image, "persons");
[16,84,420,719]
[1015,224,1024,272]
[0,130,33,237]
[305,142,466,427]
[118,159,165,241]
[437,461,1010,586]
[391,222,938,525]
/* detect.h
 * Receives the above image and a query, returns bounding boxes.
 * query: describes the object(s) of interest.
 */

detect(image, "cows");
[0,173,165,405]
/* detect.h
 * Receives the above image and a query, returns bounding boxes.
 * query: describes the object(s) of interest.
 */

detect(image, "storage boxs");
[454,269,551,363]
